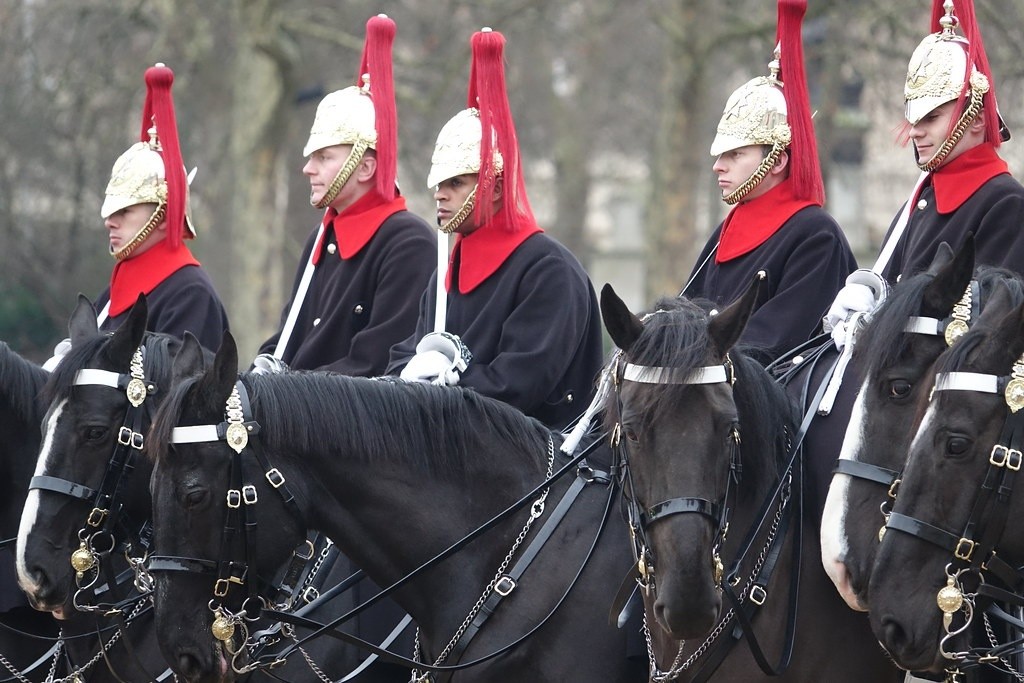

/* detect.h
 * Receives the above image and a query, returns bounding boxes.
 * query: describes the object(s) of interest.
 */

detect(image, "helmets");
[427,28,536,231]
[710,0,826,204]
[102,61,198,259]
[303,13,398,209]
[905,0,1010,171]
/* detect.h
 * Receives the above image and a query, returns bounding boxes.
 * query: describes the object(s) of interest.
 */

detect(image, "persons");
[89,141,230,354]
[823,30,1024,315]
[668,75,860,366]
[381,108,604,437]
[247,83,437,378]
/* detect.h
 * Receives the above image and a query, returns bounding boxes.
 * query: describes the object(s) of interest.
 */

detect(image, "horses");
[0,265,1023,683]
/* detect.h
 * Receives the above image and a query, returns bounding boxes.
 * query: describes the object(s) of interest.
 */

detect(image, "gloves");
[829,282,876,348]
[249,367,271,376]
[41,339,74,373]
[399,352,452,384]
